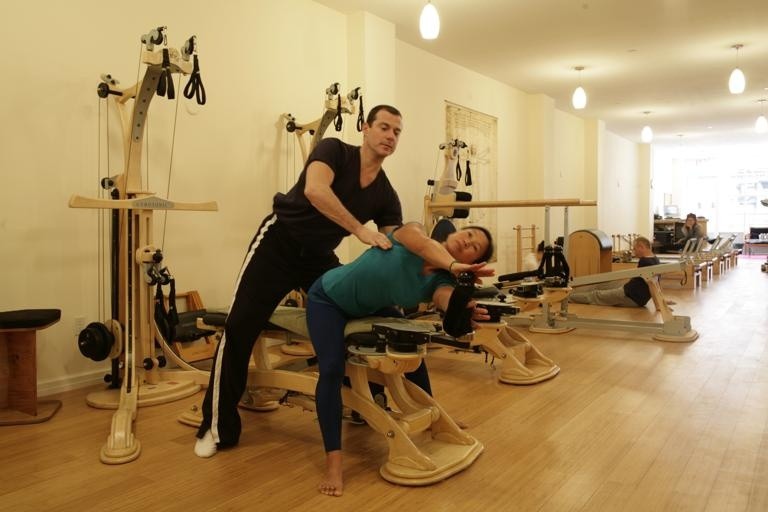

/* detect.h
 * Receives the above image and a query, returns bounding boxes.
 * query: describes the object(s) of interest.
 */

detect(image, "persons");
[522,240,544,282]
[569,238,677,313]
[194,105,402,459]
[675,213,703,252]
[308,218,495,497]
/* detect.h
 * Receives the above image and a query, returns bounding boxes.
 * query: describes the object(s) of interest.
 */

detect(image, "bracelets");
[448,259,460,271]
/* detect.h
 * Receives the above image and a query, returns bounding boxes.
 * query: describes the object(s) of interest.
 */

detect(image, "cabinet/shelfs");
[654,219,709,254]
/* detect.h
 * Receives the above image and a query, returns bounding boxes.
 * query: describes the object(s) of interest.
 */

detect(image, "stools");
[1,307,63,416]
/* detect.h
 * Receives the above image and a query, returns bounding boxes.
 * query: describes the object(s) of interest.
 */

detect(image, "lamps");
[571,64,587,109]
[755,97,768,137]
[641,109,653,144]
[727,43,748,95]
[418,0,441,39]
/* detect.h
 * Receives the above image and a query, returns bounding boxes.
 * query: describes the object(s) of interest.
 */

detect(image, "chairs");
[744,227,768,258]
